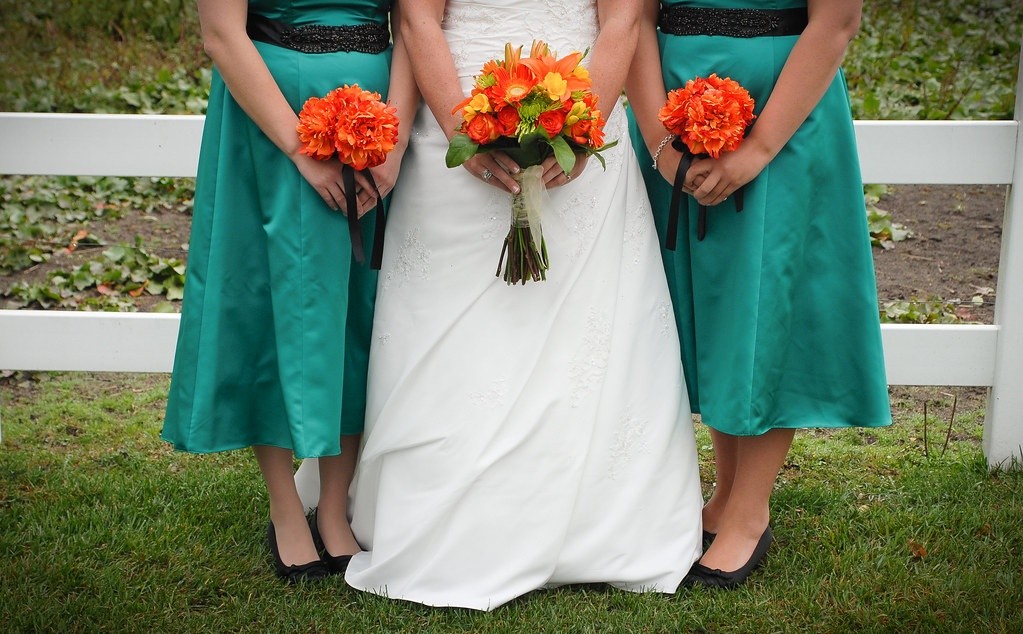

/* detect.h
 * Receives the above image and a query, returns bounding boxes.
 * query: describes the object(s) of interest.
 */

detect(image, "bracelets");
[653,128,677,172]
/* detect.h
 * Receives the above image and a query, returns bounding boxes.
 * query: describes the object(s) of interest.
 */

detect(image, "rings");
[562,170,572,182]
[482,168,492,182]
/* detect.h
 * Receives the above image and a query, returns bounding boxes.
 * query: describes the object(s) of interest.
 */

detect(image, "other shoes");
[682,526,772,589]
[701,530,718,556]
[310,506,353,574]
[268,521,322,587]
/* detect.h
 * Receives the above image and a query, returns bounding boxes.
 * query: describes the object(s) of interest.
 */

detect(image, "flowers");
[444,39,617,287]
[296,82,400,172]
[656,73,755,161]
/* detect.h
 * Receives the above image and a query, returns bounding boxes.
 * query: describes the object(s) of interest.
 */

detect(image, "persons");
[336,0,708,615]
[618,2,892,591]
[156,0,444,581]
